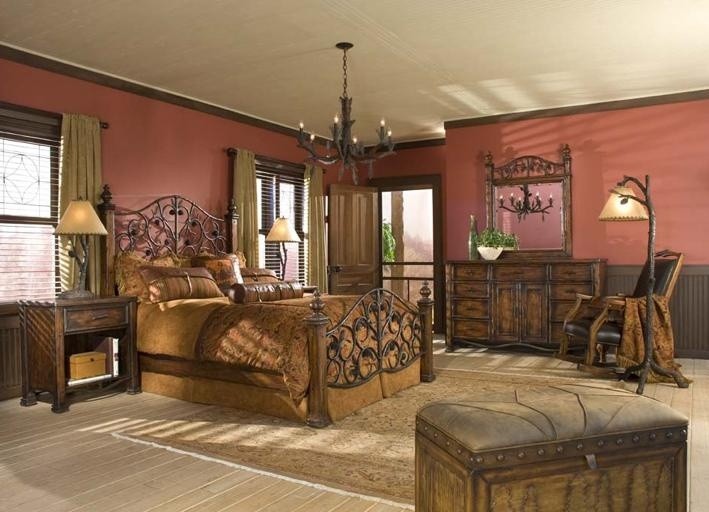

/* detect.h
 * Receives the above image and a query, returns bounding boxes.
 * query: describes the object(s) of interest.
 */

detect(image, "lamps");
[264,215,302,280]
[296,42,397,187]
[598,174,689,393]
[498,183,554,222]
[52,195,108,299]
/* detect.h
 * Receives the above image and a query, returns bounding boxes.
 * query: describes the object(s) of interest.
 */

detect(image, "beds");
[97,183,436,429]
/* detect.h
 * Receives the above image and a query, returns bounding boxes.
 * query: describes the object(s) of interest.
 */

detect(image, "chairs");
[552,247,685,376]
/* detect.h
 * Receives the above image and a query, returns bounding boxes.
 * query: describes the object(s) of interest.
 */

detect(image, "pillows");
[132,264,224,304]
[183,250,247,275]
[192,254,243,296]
[113,251,181,297]
[227,280,303,304]
[241,268,278,285]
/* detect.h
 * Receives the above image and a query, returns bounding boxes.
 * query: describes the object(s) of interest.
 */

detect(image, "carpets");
[110,368,627,511]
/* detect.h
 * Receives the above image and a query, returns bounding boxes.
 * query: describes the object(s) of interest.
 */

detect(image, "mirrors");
[484,145,573,260]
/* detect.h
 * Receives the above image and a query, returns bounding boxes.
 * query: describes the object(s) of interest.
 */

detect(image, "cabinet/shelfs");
[444,261,609,357]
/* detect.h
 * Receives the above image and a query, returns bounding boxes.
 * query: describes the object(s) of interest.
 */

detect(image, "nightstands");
[19,296,141,413]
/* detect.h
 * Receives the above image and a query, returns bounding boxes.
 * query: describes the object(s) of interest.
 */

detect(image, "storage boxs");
[414,384,690,512]
[65,351,107,381]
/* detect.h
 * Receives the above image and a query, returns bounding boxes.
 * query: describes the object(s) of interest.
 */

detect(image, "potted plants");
[502,232,520,251]
[473,228,503,259]
